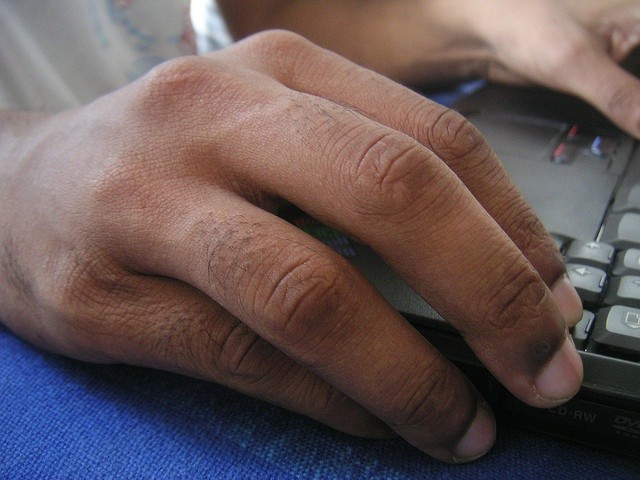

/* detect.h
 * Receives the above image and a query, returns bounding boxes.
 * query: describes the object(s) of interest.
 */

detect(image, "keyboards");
[326,49,639,402]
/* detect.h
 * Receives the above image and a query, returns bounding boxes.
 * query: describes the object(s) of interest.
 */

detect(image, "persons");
[1,1,640,463]
